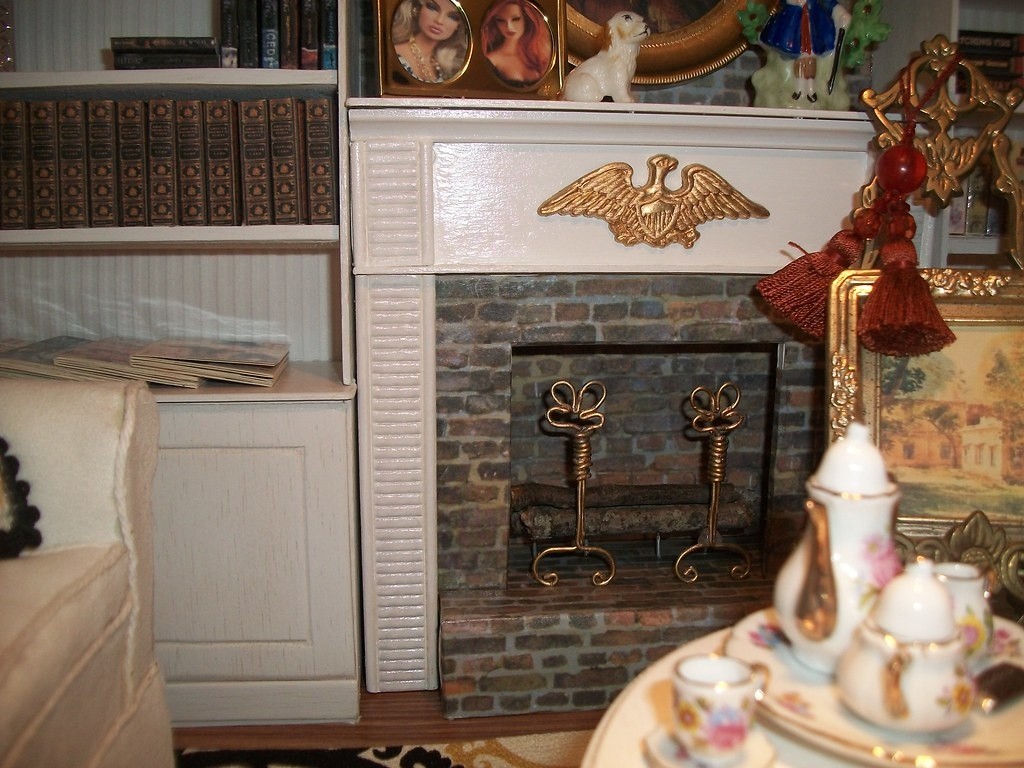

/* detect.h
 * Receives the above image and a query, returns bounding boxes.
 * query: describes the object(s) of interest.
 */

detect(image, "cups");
[668,650,770,768]
[928,560,995,664]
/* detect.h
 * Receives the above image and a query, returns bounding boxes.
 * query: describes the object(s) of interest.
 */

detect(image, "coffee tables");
[580,626,873,766]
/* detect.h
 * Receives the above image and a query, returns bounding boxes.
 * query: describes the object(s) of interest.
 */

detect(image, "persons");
[391,0,470,83]
[481,0,553,85]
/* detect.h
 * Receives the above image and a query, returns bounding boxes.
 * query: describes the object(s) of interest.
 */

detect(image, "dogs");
[560,10,649,103]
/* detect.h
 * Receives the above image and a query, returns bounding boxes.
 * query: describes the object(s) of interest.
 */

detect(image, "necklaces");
[410,37,433,78]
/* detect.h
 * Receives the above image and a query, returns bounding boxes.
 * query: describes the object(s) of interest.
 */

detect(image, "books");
[110,0,338,76]
[0,336,292,391]
[1,95,335,229]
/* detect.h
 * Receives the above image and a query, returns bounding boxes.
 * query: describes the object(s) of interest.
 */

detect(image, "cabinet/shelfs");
[140,396,365,724]
[2,2,355,389]
[875,1,1024,268]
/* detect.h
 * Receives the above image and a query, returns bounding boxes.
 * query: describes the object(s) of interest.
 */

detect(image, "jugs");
[772,421,907,678]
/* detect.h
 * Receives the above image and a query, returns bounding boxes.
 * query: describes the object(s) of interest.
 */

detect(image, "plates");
[723,604,1024,768]
[645,714,779,768]
[578,624,890,768]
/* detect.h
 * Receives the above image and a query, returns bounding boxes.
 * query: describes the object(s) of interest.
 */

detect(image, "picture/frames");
[374,0,567,102]
[828,268,1023,554]
[558,0,783,90]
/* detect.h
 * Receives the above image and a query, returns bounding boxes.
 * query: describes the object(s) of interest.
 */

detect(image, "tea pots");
[830,556,977,735]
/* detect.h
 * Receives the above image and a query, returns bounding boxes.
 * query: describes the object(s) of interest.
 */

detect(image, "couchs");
[0,372,175,768]
